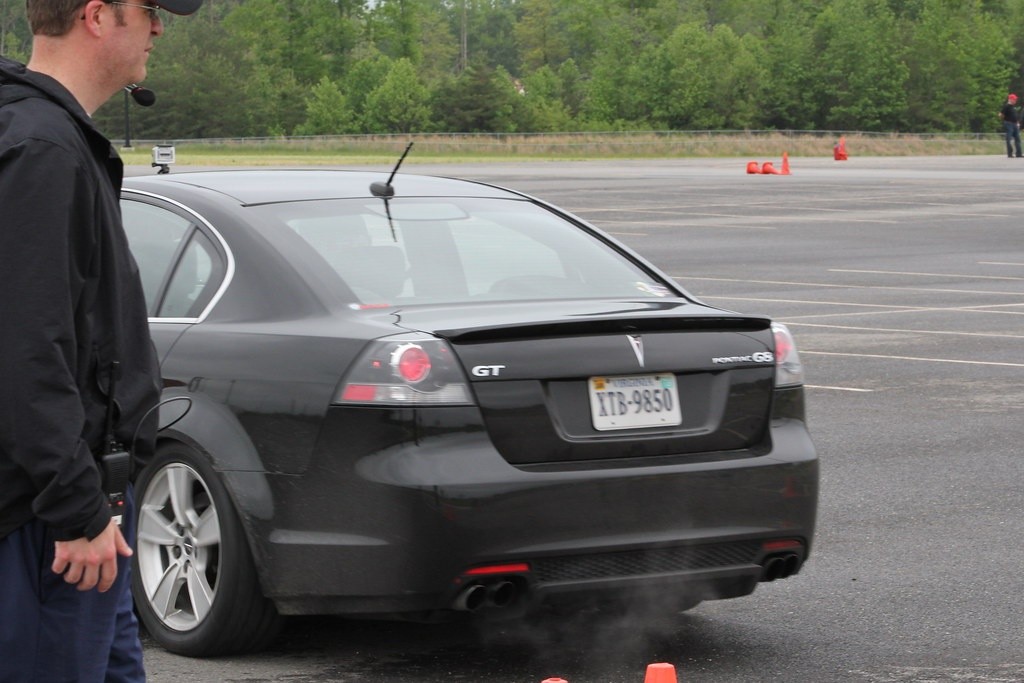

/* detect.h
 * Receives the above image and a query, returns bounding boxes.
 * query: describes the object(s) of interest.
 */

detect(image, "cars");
[115,163,820,661]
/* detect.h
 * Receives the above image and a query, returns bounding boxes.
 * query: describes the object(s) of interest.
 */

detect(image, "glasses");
[103,2,160,19]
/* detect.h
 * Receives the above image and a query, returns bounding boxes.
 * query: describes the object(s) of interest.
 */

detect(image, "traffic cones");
[778,150,792,176]
[760,160,780,175]
[835,135,849,161]
[746,160,763,177]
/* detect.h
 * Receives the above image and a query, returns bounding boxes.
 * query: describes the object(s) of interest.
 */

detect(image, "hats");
[1009,94,1018,100]
[149,0,204,15]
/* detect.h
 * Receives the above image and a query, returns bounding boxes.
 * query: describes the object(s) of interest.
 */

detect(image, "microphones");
[125,84,156,106]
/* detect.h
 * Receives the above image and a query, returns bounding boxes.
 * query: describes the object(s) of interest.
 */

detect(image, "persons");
[998,94,1024,157]
[0,1,206,683]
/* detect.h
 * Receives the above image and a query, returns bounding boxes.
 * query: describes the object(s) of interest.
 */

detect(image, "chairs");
[152,239,198,316]
[346,244,406,305]
